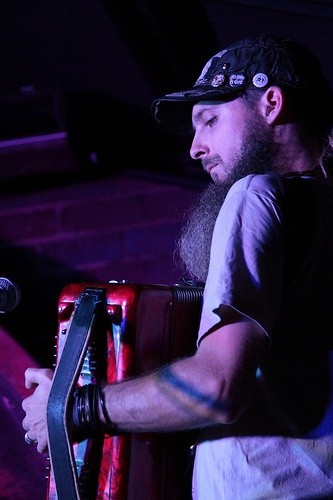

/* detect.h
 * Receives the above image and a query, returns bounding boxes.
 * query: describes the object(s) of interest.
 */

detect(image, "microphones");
[0,277,23,314]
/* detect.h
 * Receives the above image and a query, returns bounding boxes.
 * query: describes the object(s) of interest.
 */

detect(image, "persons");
[16,34,332,500]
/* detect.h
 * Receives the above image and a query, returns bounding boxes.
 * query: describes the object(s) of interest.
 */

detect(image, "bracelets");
[68,383,122,440]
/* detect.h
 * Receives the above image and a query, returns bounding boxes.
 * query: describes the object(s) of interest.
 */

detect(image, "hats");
[153,33,332,125]
